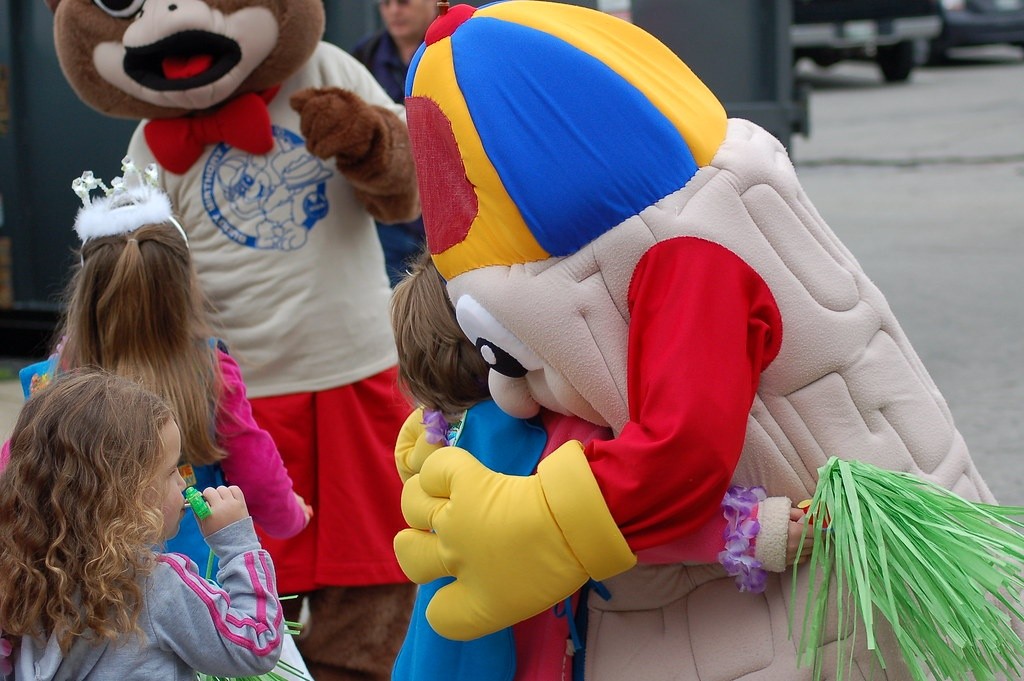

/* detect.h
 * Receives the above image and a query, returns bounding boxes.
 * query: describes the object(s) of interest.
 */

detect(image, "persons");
[0,373,285,681]
[345,0,441,286]
[391,251,826,681]
[19,156,310,584]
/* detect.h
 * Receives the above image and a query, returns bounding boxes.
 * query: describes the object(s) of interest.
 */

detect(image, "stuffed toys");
[392,0,1024,681]
[42,0,422,681]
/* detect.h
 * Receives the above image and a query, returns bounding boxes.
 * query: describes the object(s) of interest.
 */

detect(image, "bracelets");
[721,486,769,595]
